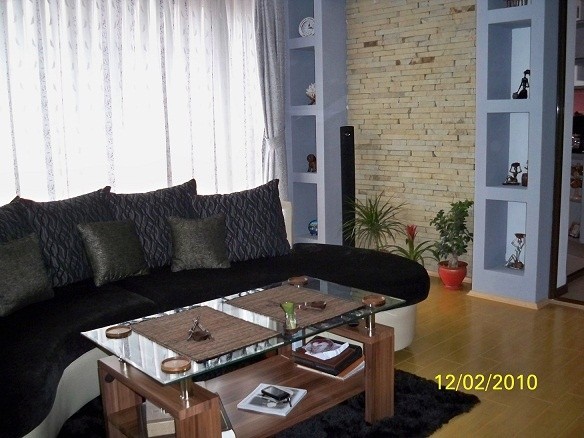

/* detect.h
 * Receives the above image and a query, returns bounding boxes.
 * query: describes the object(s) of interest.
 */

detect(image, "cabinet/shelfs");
[467,0,560,311]
[282,0,347,246]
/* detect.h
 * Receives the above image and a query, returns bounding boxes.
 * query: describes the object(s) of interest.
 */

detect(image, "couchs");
[1,177,431,438]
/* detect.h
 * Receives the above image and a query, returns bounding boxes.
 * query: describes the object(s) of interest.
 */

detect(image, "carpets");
[565,240,584,277]
[62,362,480,438]
[560,275,584,304]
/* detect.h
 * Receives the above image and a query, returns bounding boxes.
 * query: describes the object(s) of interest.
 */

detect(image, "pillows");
[184,180,292,263]
[162,212,233,273]
[0,194,33,247]
[0,232,56,319]
[109,177,198,272]
[11,185,112,289]
[73,220,152,289]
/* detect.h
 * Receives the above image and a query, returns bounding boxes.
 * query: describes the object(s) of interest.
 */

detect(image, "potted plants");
[429,199,474,291]
